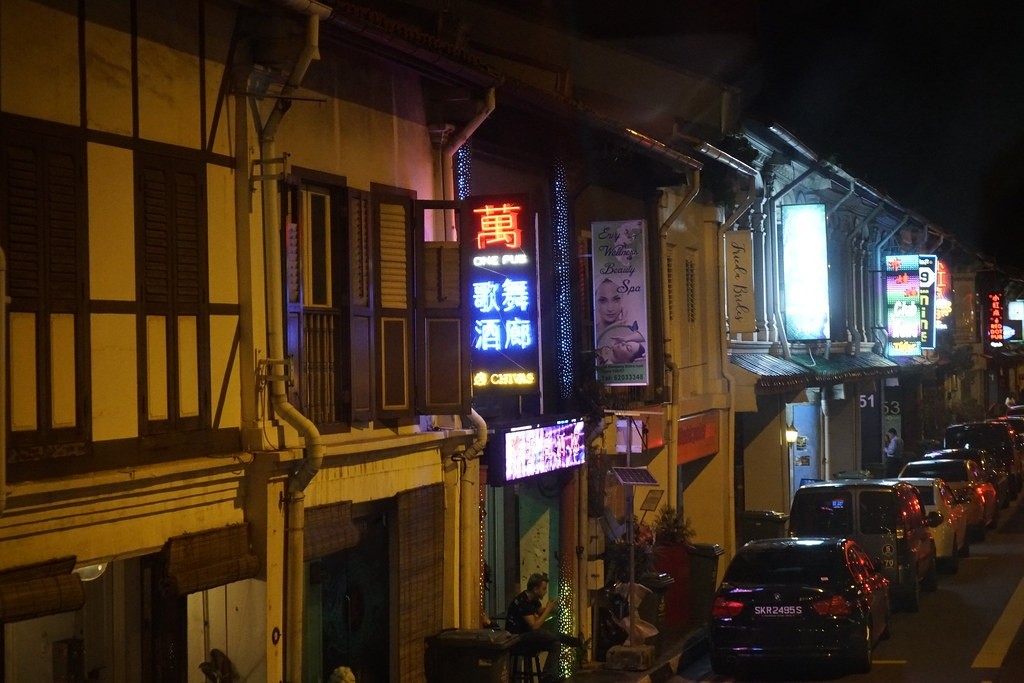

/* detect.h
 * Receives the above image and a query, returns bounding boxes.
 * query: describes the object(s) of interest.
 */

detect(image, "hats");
[330,666,355,683]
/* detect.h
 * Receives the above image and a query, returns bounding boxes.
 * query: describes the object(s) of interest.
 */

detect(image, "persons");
[596,269,645,364]
[884,428,904,477]
[505,573,583,683]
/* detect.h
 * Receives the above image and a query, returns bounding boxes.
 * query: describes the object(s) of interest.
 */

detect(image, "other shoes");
[540,676,566,683]
[578,632,583,647]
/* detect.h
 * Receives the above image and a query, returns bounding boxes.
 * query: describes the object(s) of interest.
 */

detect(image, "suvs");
[942,422,1024,501]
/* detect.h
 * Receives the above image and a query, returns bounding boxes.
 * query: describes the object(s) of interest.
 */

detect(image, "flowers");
[598,508,658,585]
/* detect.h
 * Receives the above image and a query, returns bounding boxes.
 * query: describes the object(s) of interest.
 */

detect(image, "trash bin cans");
[637,569,675,658]
[688,544,725,621]
[735,510,791,545]
[832,469,874,481]
[423,627,521,683]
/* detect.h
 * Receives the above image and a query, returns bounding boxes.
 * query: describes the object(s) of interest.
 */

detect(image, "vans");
[786,479,945,612]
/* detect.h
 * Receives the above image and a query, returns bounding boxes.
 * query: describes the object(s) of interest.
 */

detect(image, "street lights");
[602,406,663,647]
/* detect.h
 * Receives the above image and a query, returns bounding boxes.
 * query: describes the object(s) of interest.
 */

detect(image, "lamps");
[786,421,799,449]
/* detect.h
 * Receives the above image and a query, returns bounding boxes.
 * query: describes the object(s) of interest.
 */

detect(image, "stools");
[511,650,542,683]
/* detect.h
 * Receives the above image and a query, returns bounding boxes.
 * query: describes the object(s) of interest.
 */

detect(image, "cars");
[920,448,1009,508]
[896,477,971,571]
[898,459,1002,542]
[709,535,892,673]
[985,405,1024,442]
